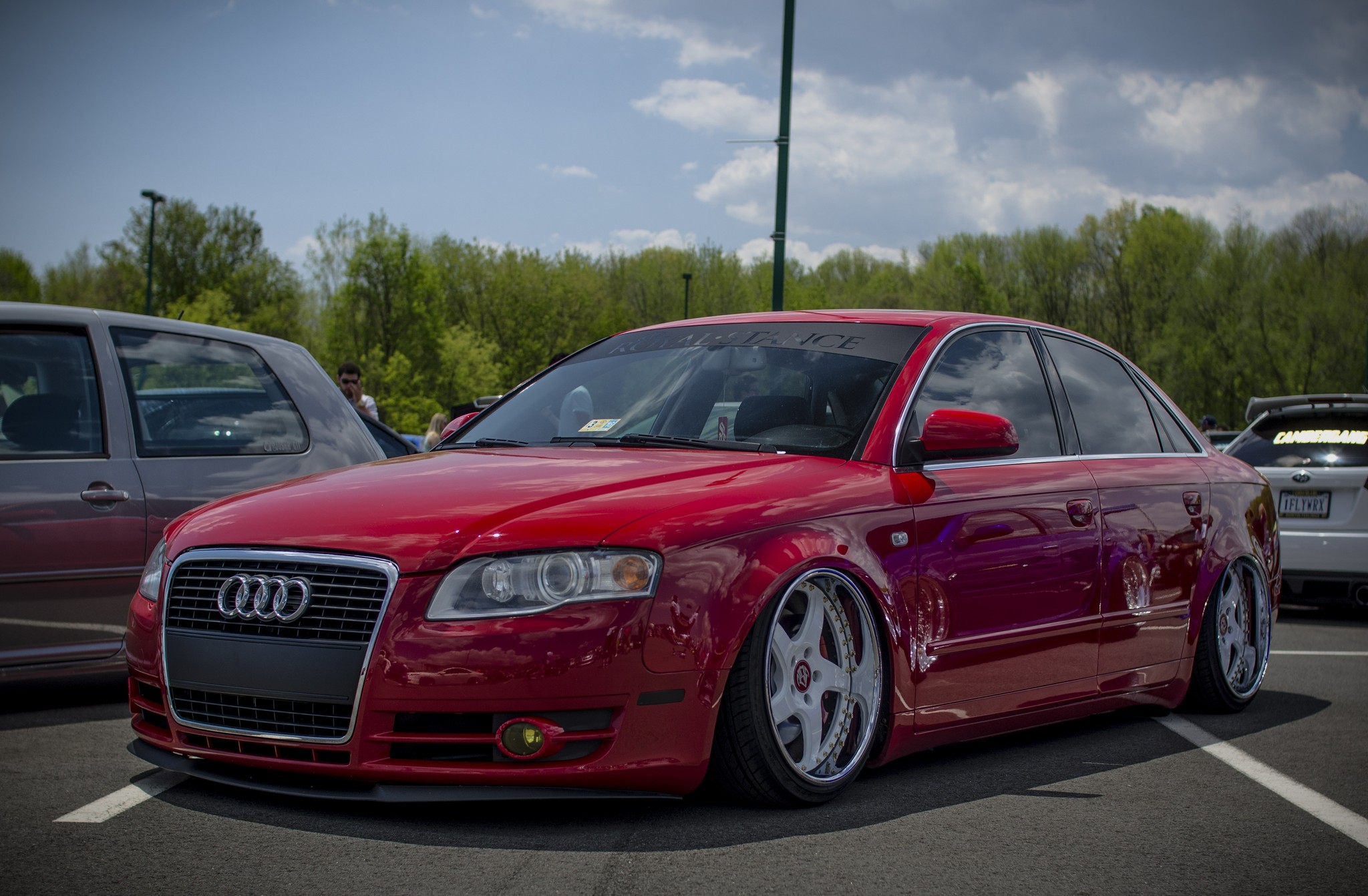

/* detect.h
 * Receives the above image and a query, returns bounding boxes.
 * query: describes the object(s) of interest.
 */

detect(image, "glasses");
[341,378,358,385]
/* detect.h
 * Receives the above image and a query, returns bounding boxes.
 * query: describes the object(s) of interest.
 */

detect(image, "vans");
[1,296,390,683]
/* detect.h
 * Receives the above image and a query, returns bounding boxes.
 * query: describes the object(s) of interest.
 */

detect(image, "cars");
[1205,393,1368,607]
[123,308,1281,821]
[133,386,422,459]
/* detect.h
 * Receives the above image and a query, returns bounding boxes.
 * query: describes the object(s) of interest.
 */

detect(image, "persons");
[1250,504,1267,548]
[422,413,448,452]
[1197,414,1231,433]
[336,363,380,422]
[1179,516,1208,596]
[542,352,594,438]
[376,594,735,707]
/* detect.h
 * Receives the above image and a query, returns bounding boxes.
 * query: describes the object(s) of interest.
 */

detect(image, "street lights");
[681,271,694,320]
[141,188,166,315]
